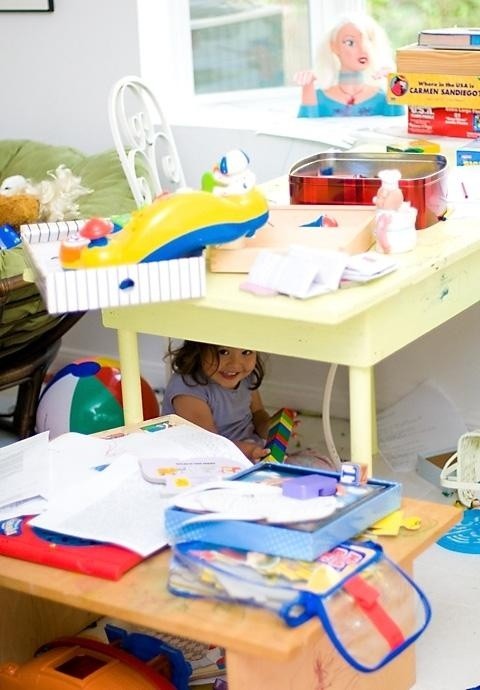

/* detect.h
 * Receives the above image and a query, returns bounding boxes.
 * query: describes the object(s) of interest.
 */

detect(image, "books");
[387,29,480,139]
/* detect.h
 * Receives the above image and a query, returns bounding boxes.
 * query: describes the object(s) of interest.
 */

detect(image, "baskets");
[441,431,480,508]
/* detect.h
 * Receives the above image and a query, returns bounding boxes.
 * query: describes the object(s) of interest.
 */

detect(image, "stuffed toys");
[0,164,94,231]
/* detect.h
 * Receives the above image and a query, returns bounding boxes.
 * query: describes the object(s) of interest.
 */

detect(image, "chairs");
[108,75,215,385]
[0,138,138,440]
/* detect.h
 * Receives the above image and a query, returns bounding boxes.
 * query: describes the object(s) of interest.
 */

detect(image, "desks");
[98,168,480,481]
[1,480,464,689]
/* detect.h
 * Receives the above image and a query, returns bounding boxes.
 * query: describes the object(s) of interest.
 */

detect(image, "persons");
[297,14,405,116]
[160,339,298,467]
